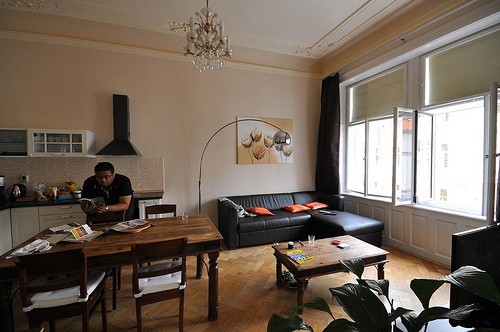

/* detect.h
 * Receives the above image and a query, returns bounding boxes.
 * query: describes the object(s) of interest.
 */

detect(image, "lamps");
[199,119,292,214]
[183,0,233,77]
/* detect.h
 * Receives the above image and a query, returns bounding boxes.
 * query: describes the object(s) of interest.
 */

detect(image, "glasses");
[96,175,113,180]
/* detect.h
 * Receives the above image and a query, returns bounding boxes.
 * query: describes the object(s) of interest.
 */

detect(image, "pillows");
[245,207,275,216]
[281,204,311,214]
[303,201,329,211]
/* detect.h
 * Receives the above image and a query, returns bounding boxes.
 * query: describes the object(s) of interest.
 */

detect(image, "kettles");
[11,183,27,198]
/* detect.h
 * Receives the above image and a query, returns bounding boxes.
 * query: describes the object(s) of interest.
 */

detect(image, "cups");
[308,235,315,247]
[288,242,294,249]
[182,208,188,221]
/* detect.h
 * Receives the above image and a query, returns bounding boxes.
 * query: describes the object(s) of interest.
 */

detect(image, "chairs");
[139,204,180,268]
[130,237,188,332]
[85,210,126,311]
[13,245,107,332]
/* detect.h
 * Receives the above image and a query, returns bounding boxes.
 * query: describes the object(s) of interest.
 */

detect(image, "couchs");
[449,222,500,332]
[217,190,385,249]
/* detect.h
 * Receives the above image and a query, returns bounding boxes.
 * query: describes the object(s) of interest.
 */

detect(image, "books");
[284,250,315,264]
[78,197,106,207]
[46,219,153,245]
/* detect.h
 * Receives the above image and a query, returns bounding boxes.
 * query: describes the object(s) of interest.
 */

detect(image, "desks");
[271,234,390,315]
[0,214,224,332]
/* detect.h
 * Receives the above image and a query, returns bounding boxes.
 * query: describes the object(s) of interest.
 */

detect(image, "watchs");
[104,206,108,213]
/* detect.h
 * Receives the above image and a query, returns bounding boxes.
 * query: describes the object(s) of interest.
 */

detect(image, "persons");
[80,162,134,221]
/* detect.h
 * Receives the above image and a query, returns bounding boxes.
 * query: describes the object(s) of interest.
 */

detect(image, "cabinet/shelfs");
[0,204,86,257]
[139,199,162,219]
[32,129,96,159]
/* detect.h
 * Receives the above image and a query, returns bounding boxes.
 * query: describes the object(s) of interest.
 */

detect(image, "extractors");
[95,94,142,156]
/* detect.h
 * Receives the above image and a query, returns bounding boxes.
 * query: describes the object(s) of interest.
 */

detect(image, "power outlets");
[15,176,18,183]
[21,176,28,183]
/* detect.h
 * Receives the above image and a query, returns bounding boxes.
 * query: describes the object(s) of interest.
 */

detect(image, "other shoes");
[281,270,299,288]
